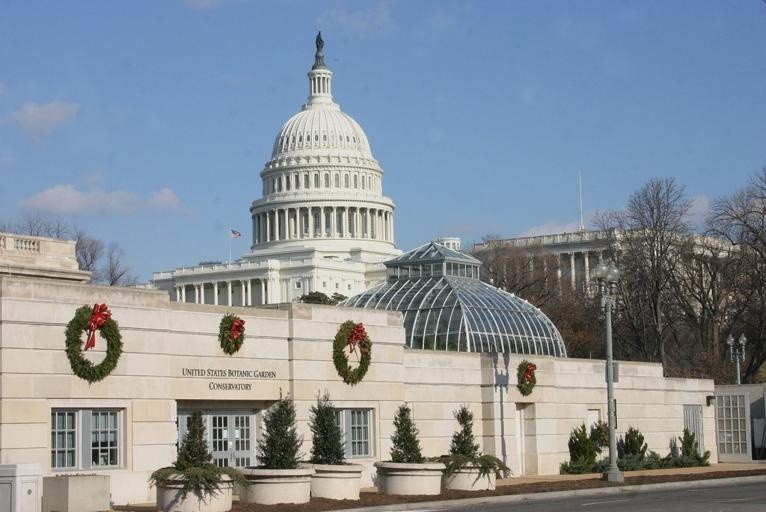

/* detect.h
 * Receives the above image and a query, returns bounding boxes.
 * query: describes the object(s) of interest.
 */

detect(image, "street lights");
[594,261,624,482]
[726,333,747,385]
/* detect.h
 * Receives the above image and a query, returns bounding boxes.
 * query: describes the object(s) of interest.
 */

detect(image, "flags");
[231,230,241,239]
